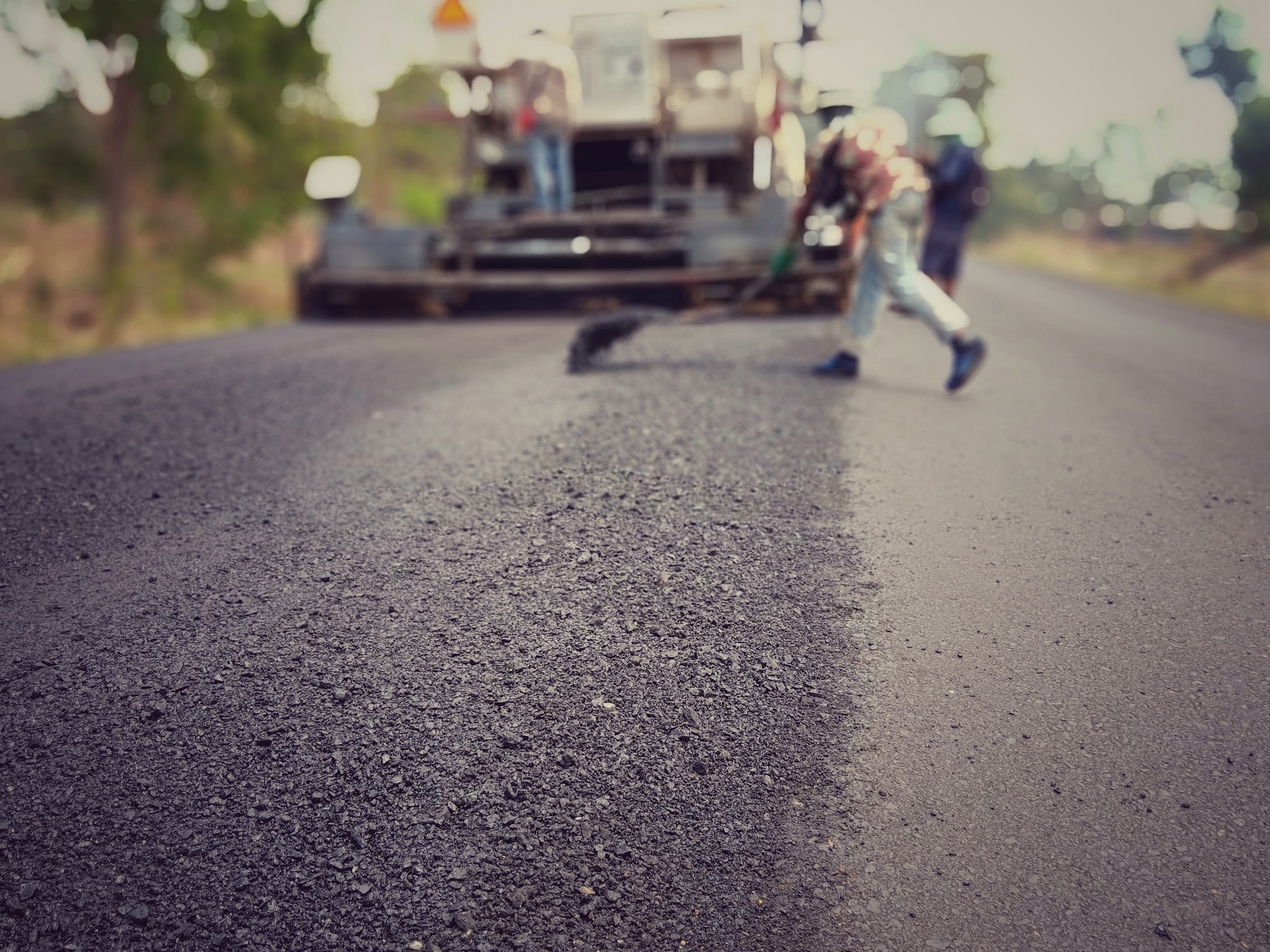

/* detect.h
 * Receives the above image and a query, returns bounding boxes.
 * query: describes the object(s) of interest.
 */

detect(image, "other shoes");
[811,350,859,377]
[946,331,988,389]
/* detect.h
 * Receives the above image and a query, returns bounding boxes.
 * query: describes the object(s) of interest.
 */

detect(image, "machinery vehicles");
[290,3,864,329]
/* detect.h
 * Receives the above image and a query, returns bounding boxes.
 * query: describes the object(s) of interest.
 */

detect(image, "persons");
[764,86,991,393]
[884,109,978,318]
